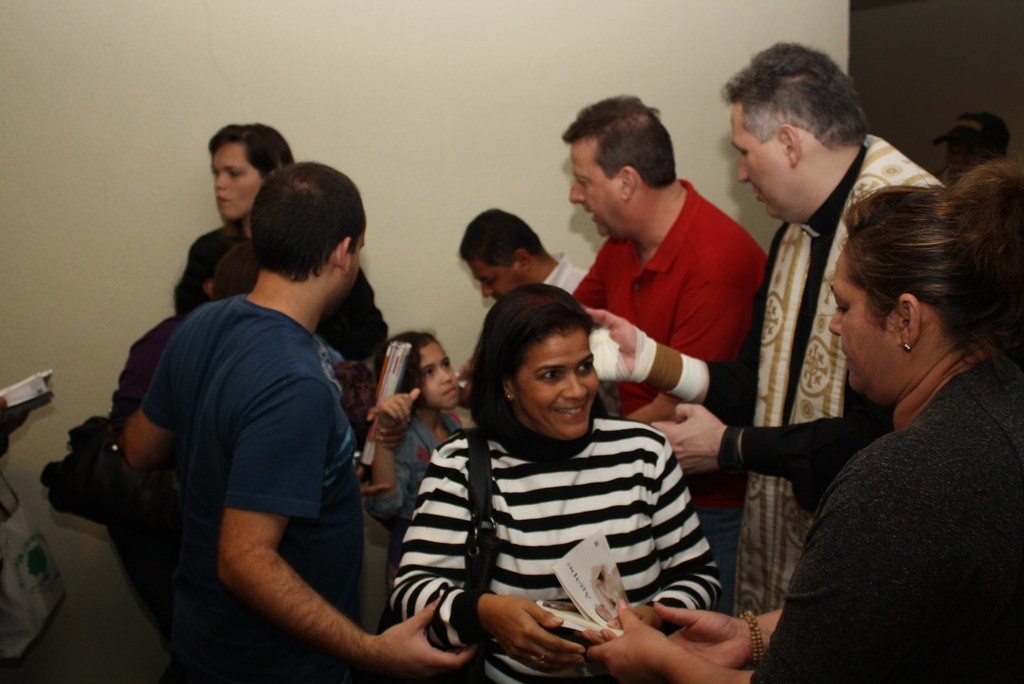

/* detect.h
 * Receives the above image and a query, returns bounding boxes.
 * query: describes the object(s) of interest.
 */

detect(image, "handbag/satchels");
[371,427,499,684]
[0,470,65,658]
[41,417,184,539]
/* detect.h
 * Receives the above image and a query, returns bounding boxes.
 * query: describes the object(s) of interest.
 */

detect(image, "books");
[0,369,54,409]
[362,340,412,465]
[537,533,629,637]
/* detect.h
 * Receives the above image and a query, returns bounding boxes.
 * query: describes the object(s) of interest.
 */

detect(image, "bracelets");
[738,614,763,666]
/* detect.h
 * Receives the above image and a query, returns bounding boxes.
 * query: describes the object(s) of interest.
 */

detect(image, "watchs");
[718,424,743,475]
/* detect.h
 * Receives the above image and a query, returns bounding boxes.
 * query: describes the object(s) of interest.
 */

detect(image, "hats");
[932,112,1010,157]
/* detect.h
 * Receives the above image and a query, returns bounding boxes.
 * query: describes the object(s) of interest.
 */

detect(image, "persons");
[562,96,767,616]
[367,330,460,636]
[457,208,589,408]
[387,284,722,684]
[584,42,943,619]
[175,123,389,450]
[932,112,1011,186]
[591,564,629,622]
[124,163,477,684]
[0,397,29,460]
[589,164,1024,684]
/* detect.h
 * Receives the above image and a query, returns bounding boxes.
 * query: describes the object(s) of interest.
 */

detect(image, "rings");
[530,652,546,666]
